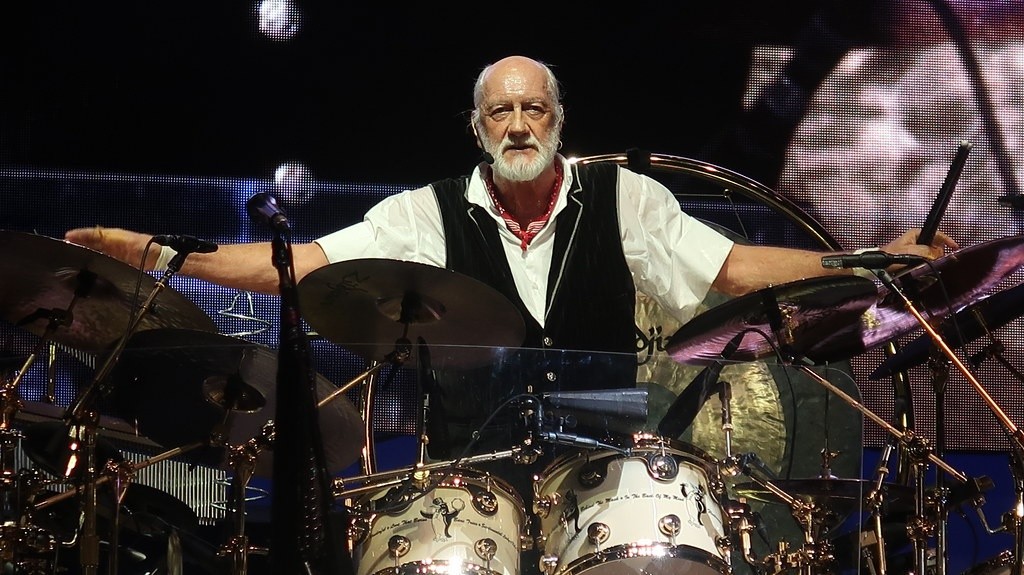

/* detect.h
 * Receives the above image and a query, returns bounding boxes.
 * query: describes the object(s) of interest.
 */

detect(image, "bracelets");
[153,245,177,272]
[850,247,882,281]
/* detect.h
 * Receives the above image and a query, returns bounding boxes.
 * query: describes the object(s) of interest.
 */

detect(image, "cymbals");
[93,322,370,478]
[866,279,1024,382]
[731,474,913,507]
[0,222,224,357]
[291,254,529,373]
[661,265,880,365]
[843,230,1024,352]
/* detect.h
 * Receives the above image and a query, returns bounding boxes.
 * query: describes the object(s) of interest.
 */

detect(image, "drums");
[350,461,531,575]
[528,431,734,575]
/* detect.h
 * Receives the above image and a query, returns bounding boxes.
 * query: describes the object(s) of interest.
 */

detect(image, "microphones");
[474,124,494,164]
[248,192,289,228]
[152,234,219,254]
[658,332,745,440]
[417,337,436,385]
[822,253,926,269]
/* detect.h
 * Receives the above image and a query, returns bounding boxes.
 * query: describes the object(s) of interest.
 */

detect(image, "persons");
[61,54,959,463]
